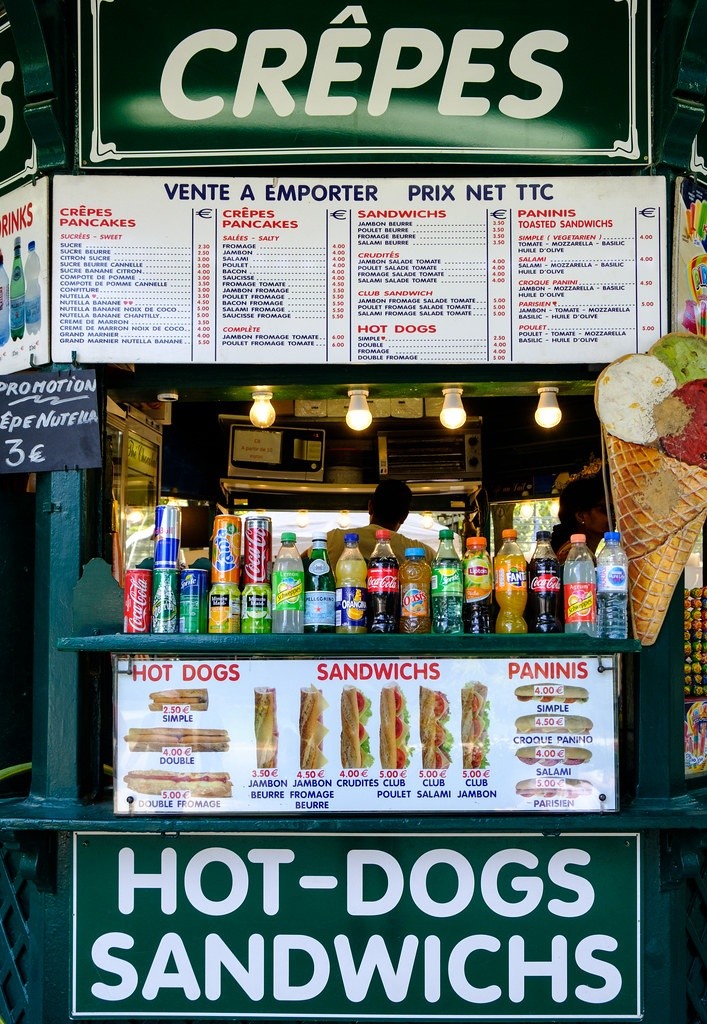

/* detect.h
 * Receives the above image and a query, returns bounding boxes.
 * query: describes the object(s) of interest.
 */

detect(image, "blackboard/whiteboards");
[0,369,104,472]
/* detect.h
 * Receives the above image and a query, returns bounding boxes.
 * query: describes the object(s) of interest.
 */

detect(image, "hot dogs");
[255,681,492,770]
[514,684,596,798]
[124,689,234,798]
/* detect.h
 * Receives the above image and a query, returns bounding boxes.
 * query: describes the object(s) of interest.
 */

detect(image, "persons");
[298,480,437,572]
[548,477,608,566]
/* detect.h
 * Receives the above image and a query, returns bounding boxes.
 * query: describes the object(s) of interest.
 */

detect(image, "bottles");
[271,533,304,633]
[431,530,463,633]
[366,530,399,633]
[398,548,430,634]
[0,249,9,347]
[564,533,597,637]
[463,537,494,634]
[336,534,367,634]
[304,532,335,633]
[24,241,41,334]
[529,531,561,634]
[493,529,528,633]
[596,532,628,640]
[9,236,25,341]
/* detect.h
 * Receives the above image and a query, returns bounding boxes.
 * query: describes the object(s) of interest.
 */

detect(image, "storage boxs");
[133,401,172,425]
[271,397,445,418]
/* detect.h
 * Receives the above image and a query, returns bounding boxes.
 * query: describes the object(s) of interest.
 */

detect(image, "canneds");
[122,505,272,634]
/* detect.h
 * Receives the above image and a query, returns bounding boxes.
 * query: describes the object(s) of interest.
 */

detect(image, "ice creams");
[596,332,707,645]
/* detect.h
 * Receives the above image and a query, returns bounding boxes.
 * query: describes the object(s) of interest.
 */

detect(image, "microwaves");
[228,424,325,482]
